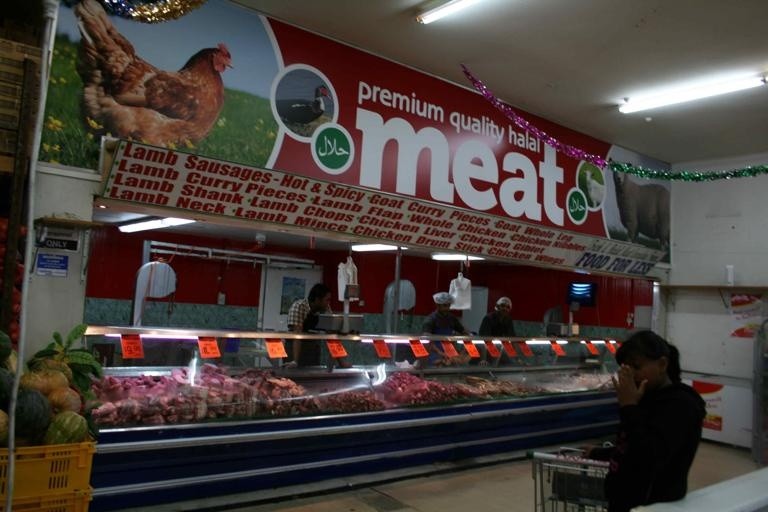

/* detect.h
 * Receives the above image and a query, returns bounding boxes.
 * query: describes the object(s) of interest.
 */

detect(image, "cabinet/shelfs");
[82,325,623,512]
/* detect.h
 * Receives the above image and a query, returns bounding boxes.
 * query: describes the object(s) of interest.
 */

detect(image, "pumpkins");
[0,331,88,445]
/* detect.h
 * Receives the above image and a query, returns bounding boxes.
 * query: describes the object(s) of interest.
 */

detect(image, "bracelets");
[292,360,298,367]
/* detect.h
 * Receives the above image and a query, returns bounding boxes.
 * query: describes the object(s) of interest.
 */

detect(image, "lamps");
[618,71,768,114]
[416,0,478,24]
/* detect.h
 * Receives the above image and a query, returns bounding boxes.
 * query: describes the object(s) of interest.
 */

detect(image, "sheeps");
[609,157,670,252]
[582,169,606,208]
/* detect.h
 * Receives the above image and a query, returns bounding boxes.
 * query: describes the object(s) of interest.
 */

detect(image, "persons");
[478,295,516,366]
[419,291,475,366]
[603,330,707,512]
[280,280,350,370]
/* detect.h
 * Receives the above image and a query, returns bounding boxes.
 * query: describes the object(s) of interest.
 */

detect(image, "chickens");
[73,0,235,154]
[275,84,333,128]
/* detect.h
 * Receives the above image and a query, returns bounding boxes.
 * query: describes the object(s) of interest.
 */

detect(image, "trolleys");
[526,441,614,512]
